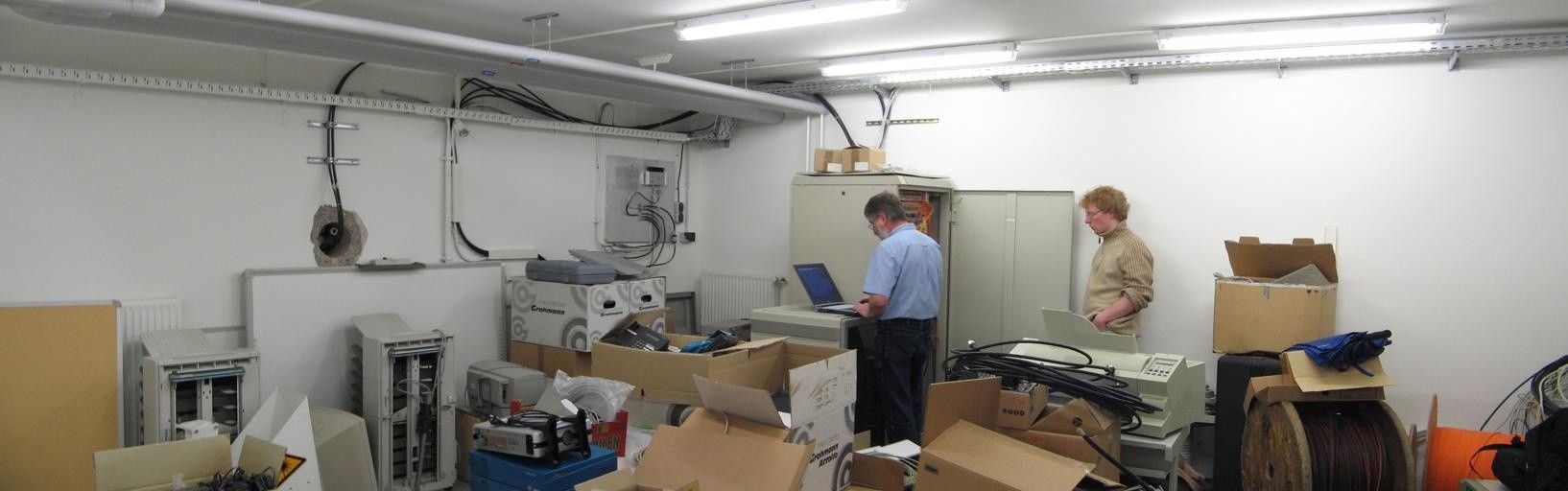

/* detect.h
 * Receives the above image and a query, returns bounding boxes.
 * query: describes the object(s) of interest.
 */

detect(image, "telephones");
[600,313,668,351]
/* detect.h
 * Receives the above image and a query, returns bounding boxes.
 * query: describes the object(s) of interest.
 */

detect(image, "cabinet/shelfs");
[344,338,457,491]
[788,172,1076,375]
[134,350,256,446]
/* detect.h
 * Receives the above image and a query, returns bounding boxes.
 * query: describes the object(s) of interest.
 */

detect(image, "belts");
[885,319,930,328]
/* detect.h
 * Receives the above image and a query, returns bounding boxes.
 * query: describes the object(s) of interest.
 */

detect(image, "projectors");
[473,411,577,459]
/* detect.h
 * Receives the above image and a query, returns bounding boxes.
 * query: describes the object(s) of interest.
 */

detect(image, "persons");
[1078,184,1154,339]
[848,190,946,460]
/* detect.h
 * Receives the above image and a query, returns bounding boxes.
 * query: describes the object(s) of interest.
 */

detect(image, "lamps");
[108,0,1566,124]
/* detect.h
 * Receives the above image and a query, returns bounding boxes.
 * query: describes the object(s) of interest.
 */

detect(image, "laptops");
[792,260,862,317]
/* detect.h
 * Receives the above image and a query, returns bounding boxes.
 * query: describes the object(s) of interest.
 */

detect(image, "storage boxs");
[471,273,1133,487]
[1209,236,1342,358]
[94,434,289,489]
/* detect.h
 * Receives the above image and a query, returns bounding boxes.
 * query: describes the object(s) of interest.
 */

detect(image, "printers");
[1009,308,1209,440]
[466,359,554,415]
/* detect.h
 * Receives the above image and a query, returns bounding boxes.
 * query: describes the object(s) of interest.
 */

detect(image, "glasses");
[1084,210,1103,220]
[867,216,882,231]
